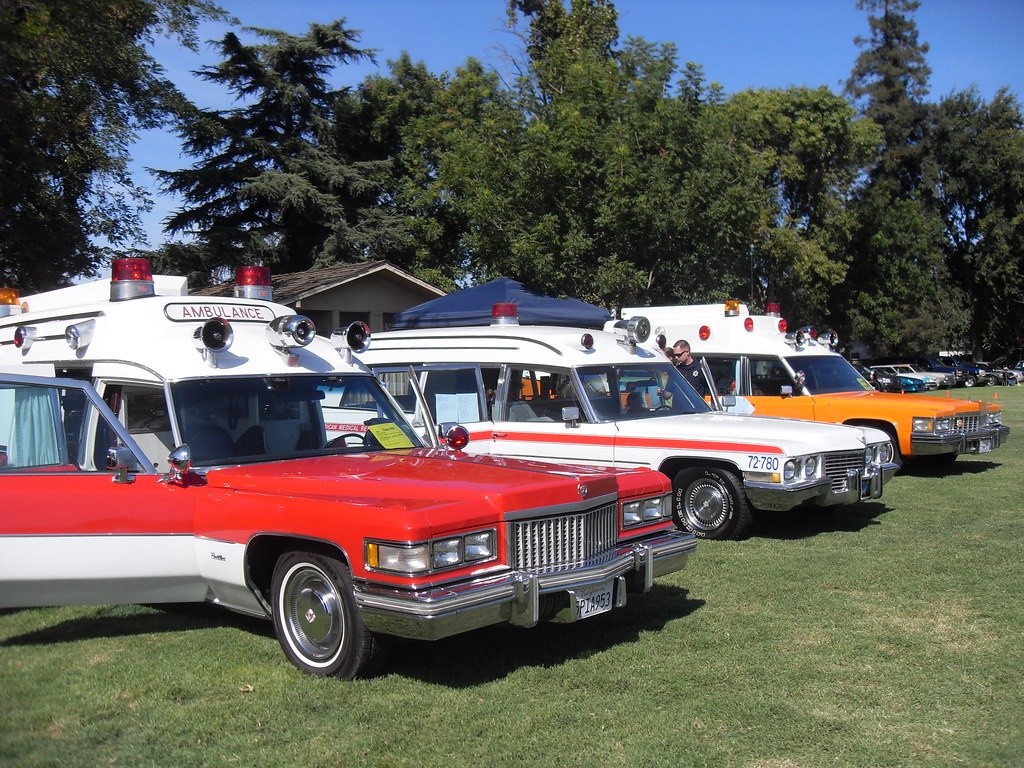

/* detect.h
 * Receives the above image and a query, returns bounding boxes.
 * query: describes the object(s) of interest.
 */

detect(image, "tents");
[393,277,612,330]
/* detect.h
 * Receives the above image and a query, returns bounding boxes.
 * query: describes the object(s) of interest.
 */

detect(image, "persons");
[624,392,650,415]
[656,340,706,412]
[1002,367,1022,387]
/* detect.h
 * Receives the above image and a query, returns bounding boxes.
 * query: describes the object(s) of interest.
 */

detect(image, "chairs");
[183,425,236,462]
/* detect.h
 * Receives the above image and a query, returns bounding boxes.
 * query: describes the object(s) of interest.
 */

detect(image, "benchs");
[491,397,620,422]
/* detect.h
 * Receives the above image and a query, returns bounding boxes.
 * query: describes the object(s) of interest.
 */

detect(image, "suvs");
[584,300,1010,468]
[310,301,902,544]
[0,258,699,685]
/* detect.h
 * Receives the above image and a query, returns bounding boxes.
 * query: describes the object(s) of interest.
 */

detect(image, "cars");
[851,356,1023,393]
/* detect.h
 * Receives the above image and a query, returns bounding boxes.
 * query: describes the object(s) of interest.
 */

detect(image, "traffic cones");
[993,391,999,400]
[944,390,951,398]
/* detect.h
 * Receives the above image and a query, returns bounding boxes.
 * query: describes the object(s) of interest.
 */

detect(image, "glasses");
[674,350,686,358]
